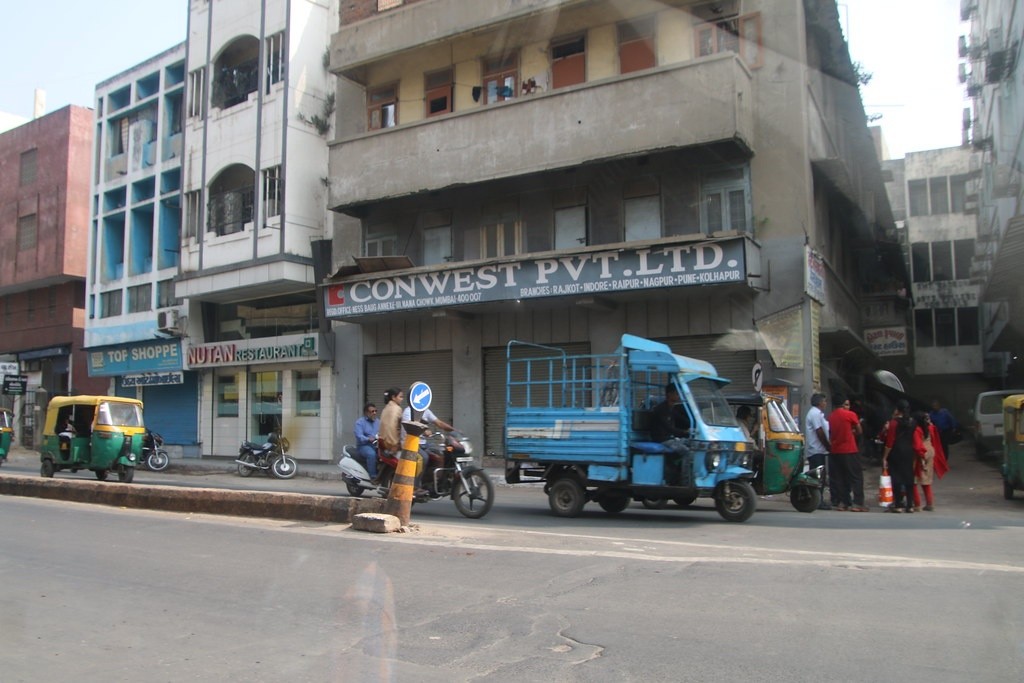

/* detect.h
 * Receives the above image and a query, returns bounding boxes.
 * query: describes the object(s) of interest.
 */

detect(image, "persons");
[929,401,955,460]
[58,416,76,446]
[806,393,830,470]
[401,407,464,496]
[378,387,403,449]
[879,399,936,513]
[828,392,869,512]
[654,384,690,481]
[354,404,380,485]
[736,406,758,437]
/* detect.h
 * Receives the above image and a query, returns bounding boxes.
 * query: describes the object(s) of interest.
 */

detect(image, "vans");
[969,389,1024,458]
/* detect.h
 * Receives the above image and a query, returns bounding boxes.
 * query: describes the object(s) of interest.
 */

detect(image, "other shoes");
[907,507,913,513]
[838,505,847,511]
[819,502,832,510]
[414,488,429,497]
[852,505,869,512]
[371,478,379,485]
[914,508,921,512]
[891,507,902,513]
[923,507,932,511]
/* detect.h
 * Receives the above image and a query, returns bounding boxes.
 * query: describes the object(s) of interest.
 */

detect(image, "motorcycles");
[371,430,495,519]
[669,391,824,514]
[235,418,298,480]
[998,393,1024,500]
[40,395,146,483]
[502,333,759,525]
[0,407,16,464]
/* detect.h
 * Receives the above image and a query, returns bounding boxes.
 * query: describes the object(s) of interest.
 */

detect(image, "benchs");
[630,410,676,456]
[70,437,92,451]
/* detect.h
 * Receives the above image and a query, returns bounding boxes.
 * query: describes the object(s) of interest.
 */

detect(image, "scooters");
[140,426,169,471]
[336,432,385,497]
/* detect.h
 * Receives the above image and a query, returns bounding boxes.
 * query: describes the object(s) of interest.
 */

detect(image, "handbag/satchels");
[948,430,963,445]
[879,468,894,507]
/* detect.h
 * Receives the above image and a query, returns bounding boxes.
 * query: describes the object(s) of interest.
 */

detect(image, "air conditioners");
[156,309,179,329]
[987,26,1006,81]
